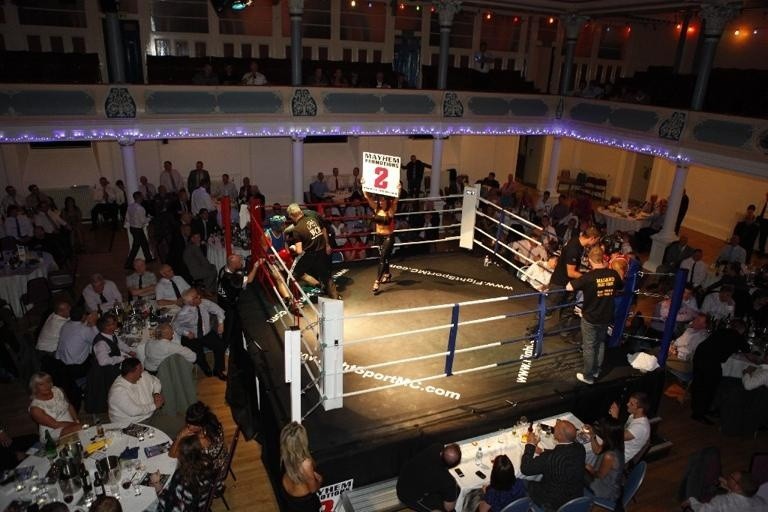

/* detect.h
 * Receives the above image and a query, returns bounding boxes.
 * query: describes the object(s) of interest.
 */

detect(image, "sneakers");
[373,280,380,291]
[381,274,391,283]
[577,373,593,385]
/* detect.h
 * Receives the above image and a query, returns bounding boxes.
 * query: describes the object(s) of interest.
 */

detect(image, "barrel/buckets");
[96,455,123,486]
[57,462,82,494]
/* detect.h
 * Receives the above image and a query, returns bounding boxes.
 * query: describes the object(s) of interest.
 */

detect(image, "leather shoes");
[214,370,225,379]
[203,367,211,376]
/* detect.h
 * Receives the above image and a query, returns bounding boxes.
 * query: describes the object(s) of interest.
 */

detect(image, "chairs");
[1,193,255,406]
[437,407,767,511]
[0,407,241,511]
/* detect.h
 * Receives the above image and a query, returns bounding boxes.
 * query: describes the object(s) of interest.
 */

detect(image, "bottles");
[92,296,160,330]
[706,311,767,356]
[473,416,597,466]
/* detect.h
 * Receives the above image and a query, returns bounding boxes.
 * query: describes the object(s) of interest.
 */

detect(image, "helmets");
[602,234,623,255]
[270,215,286,232]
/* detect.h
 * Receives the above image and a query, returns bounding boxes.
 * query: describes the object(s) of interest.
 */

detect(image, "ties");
[197,306,203,341]
[100,293,107,303]
[171,280,181,298]
[138,276,142,301]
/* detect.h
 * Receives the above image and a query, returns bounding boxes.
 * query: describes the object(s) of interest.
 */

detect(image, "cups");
[13,422,154,504]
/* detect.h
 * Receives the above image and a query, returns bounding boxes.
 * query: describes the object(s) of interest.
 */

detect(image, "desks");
[578,250,768,394]
[318,176,660,240]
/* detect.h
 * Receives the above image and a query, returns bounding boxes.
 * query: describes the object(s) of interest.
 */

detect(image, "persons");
[28,371,82,445]
[617,87,632,102]
[149,435,215,510]
[585,427,621,502]
[549,192,595,239]
[280,421,323,510]
[219,65,240,85]
[449,172,558,273]
[479,454,527,511]
[678,444,766,511]
[731,203,759,265]
[33,257,228,401]
[2,426,40,471]
[263,201,343,316]
[217,253,265,352]
[108,357,183,441]
[196,65,221,85]
[240,62,271,85]
[520,420,586,510]
[632,188,689,255]
[569,79,592,99]
[168,400,229,499]
[609,391,650,462]
[632,88,651,105]
[371,72,391,88]
[308,68,330,87]
[645,233,766,436]
[90,160,261,296]
[312,154,432,292]
[396,441,461,510]
[2,183,83,269]
[399,79,416,89]
[348,70,368,88]
[594,81,623,101]
[523,227,641,383]
[330,67,349,86]
